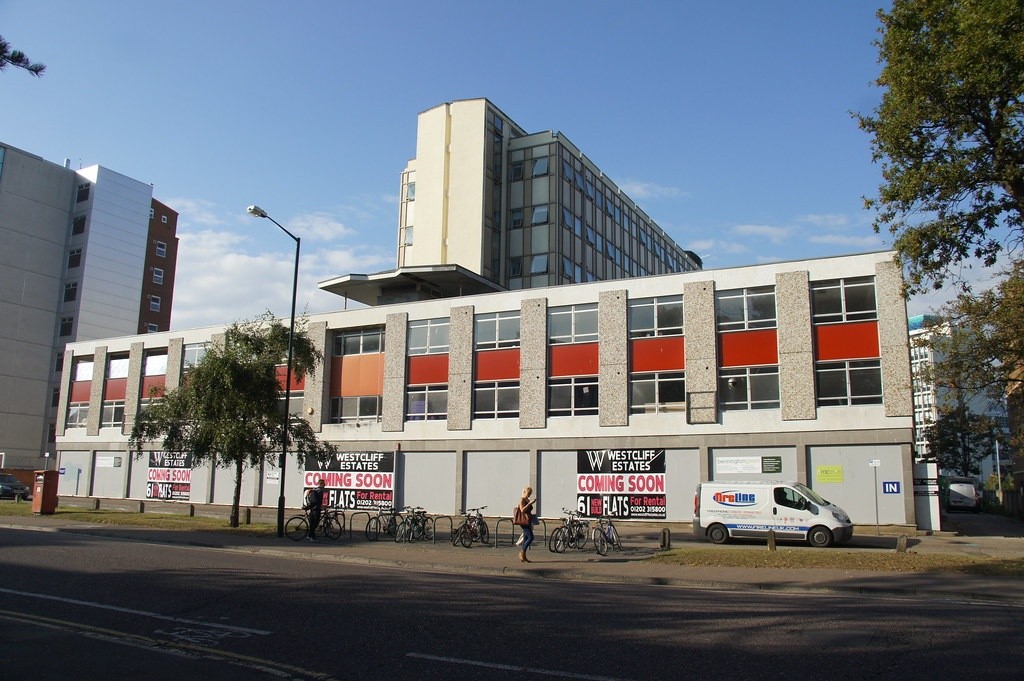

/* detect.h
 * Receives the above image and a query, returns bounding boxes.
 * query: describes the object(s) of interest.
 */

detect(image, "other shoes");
[519,553,530,563]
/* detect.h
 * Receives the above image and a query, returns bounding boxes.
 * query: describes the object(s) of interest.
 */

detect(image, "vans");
[691,480,853,547]
[945,479,979,515]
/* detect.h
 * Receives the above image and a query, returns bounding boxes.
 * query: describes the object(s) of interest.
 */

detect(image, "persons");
[517,488,538,563]
[778,492,797,506]
[306,479,325,543]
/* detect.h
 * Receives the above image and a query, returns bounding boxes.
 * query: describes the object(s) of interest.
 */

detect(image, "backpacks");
[305,487,321,508]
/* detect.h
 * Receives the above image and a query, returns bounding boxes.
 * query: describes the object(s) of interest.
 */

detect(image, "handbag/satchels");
[515,533,525,546]
[513,498,532,525]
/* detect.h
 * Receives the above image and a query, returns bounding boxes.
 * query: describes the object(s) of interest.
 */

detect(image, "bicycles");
[285,505,625,557]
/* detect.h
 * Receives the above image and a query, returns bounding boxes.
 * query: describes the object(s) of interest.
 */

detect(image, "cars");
[0,473,30,500]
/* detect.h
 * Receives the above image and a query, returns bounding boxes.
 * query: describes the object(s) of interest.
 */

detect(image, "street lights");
[245,203,302,538]
